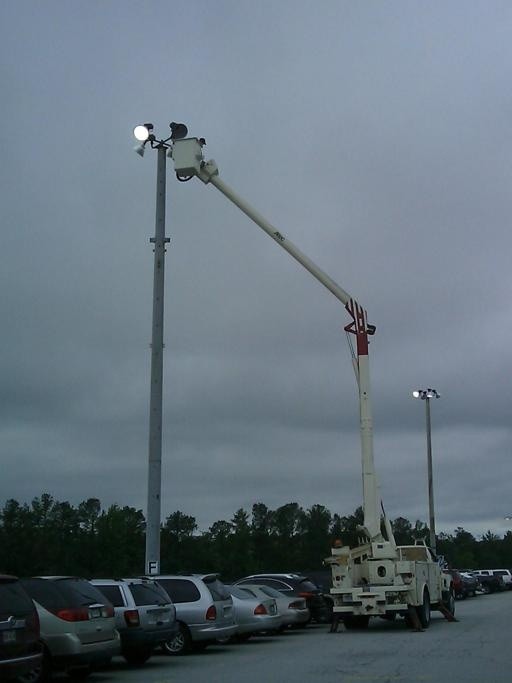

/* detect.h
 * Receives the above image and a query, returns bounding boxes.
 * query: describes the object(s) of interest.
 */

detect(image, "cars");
[250,583,311,636]
[217,581,284,645]
[453,567,512,599]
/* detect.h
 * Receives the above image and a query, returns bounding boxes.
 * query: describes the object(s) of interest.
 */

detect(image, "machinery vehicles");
[175,139,461,633]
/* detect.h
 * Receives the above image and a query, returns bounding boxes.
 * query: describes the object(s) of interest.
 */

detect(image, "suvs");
[231,570,328,622]
[0,571,53,682]
[21,571,123,681]
[147,570,241,655]
[84,572,178,670]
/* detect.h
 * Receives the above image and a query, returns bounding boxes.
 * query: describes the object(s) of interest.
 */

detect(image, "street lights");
[124,113,192,575]
[503,514,512,520]
[411,387,443,557]
